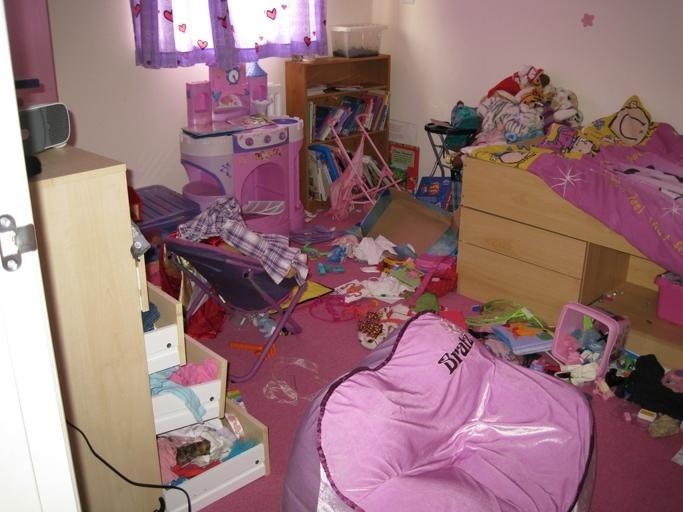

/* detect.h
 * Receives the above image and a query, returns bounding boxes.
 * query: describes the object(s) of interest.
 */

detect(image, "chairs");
[162,229,309,386]
[424,100,478,179]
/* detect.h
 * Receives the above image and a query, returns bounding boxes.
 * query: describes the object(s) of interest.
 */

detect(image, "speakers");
[18,102,71,156]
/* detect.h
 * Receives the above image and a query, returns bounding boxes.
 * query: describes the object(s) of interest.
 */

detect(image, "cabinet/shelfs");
[28,146,273,512]
[285,58,391,212]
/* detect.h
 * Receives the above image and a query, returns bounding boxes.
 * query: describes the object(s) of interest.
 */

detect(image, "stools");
[128,184,201,251]
[282,309,598,512]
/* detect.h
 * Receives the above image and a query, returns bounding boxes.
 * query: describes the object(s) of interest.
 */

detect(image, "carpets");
[147,235,683,512]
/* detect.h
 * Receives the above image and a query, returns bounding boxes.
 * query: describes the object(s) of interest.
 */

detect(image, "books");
[306,81,390,211]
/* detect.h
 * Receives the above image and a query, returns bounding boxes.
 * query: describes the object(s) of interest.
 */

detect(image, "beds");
[454,137,683,387]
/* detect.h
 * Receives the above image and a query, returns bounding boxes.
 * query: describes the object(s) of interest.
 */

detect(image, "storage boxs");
[328,23,388,59]
[654,271,683,325]
